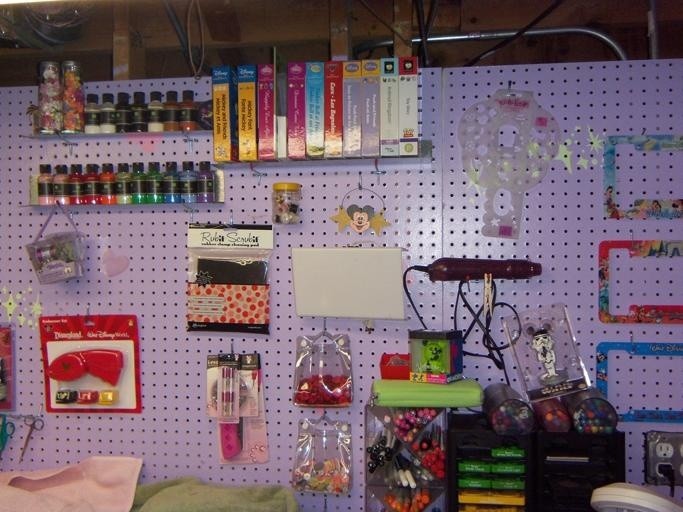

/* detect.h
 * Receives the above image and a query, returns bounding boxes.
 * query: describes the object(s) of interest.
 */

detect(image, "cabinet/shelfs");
[364,403,626,511]
[18,128,226,208]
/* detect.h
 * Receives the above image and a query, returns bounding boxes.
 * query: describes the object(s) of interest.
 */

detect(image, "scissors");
[0,414,15,454]
[18,415,44,464]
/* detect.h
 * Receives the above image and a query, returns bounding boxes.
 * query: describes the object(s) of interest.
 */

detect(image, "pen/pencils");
[484,384,617,434]
[222,367,234,416]
[365,406,448,512]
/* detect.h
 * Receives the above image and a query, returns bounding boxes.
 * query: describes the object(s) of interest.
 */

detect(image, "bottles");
[36,161,217,206]
[271,182,301,226]
[38,59,199,135]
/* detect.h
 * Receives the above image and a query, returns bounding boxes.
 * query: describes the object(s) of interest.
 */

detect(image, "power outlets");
[645,429,682,486]
